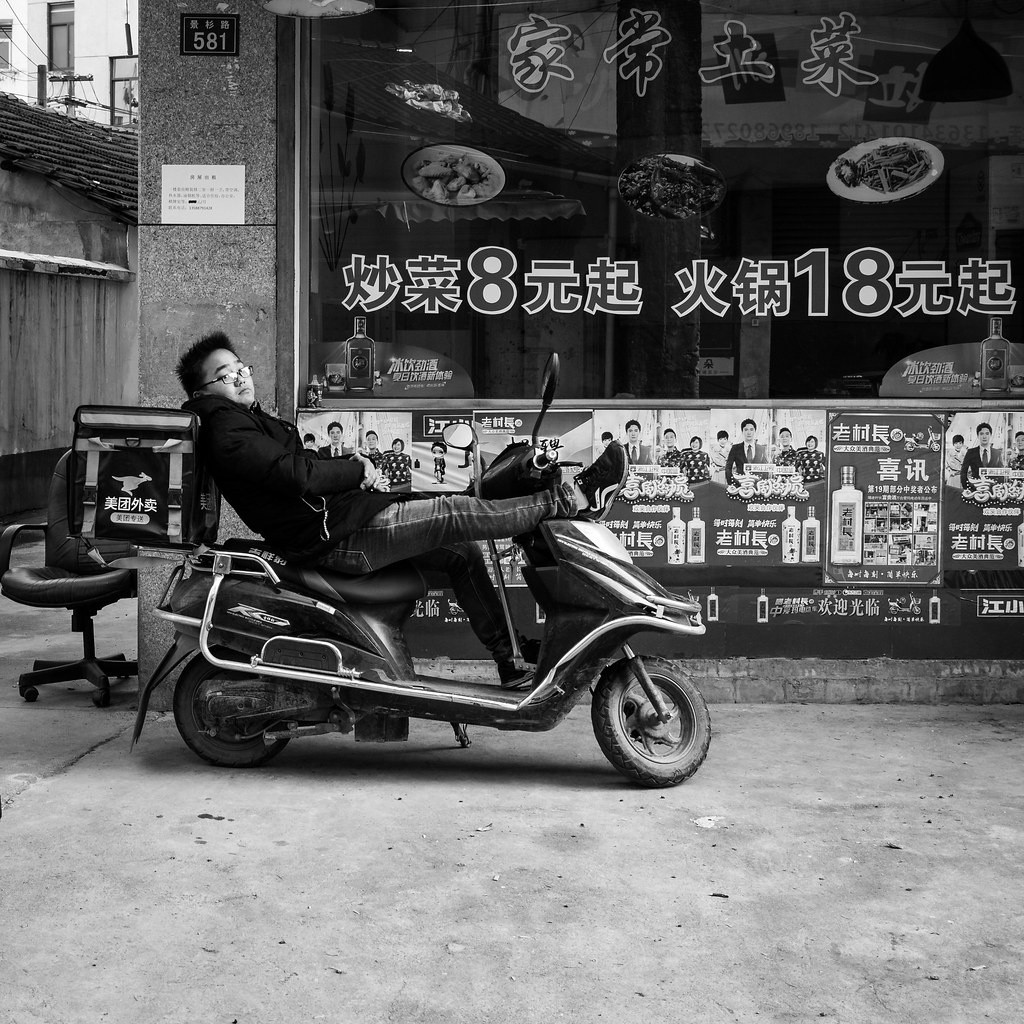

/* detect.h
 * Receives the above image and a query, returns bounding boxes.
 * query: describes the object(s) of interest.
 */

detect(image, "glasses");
[195,364,254,393]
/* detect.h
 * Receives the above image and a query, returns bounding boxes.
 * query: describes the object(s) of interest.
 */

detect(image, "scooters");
[120,352,719,791]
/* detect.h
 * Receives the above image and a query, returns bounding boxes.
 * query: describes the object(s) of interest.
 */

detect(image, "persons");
[298,419,1024,522]
[171,332,629,690]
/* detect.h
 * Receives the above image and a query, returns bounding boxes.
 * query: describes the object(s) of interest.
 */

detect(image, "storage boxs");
[65,404,220,551]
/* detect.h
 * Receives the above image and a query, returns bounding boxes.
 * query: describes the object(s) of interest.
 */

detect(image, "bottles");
[801,506,820,562]
[830,465,863,565]
[929,589,940,624]
[345,316,375,391]
[781,506,801,564]
[980,318,1011,391]
[757,588,768,623]
[707,586,719,621]
[667,507,686,564]
[310,375,319,384]
[687,507,705,563]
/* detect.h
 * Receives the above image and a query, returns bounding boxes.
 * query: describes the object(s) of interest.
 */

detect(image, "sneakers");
[498,636,542,689]
[574,439,629,523]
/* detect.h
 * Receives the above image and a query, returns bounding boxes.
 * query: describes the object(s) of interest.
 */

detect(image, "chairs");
[0,448,140,708]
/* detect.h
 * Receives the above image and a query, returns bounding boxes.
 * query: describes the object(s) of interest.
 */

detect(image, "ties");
[747,445,752,463]
[982,449,989,467]
[334,448,339,457]
[632,446,638,464]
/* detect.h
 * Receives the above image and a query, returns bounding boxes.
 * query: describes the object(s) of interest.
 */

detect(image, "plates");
[619,152,726,223]
[827,137,945,205]
[400,142,506,206]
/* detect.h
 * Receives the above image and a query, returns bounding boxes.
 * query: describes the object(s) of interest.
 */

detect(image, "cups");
[325,363,348,391]
